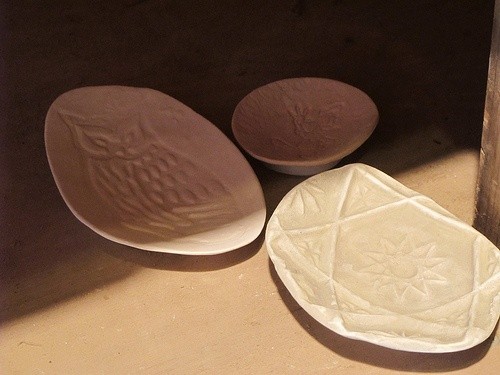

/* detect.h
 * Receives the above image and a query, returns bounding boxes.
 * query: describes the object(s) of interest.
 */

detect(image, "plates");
[265,164,500,353]
[44,86,266,256]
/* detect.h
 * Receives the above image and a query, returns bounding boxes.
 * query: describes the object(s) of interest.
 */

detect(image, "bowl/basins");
[230,79,379,176]
